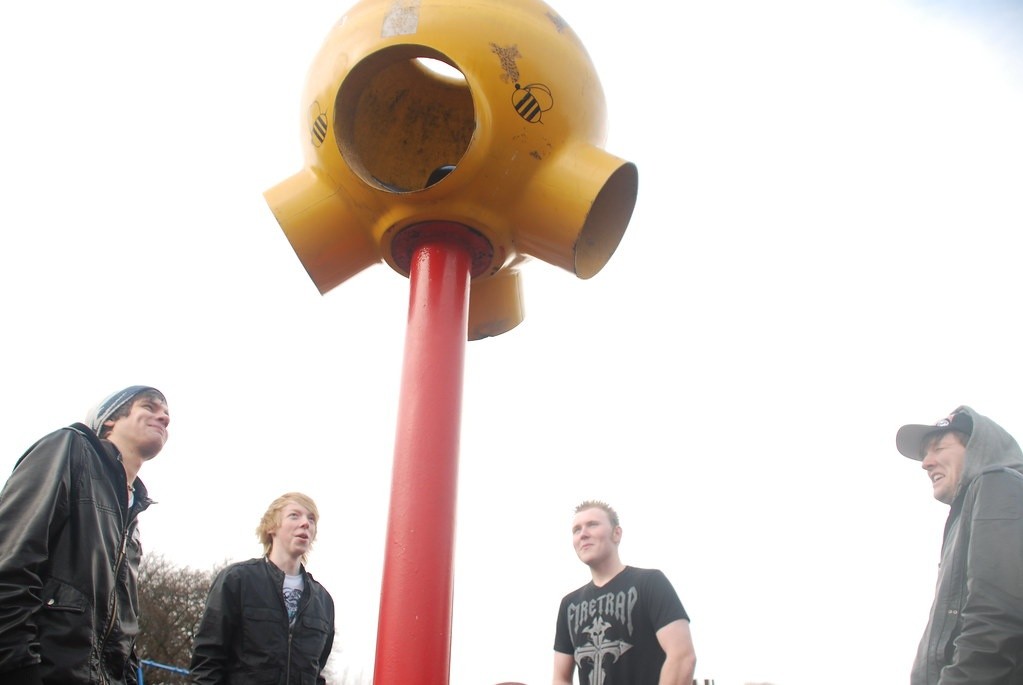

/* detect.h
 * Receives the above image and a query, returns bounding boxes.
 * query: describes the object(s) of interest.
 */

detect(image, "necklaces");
[127,482,135,493]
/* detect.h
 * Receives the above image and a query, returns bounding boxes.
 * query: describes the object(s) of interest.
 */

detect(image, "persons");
[190,493,335,685]
[551,501,696,685]
[0,385,170,685]
[896,406,1023,685]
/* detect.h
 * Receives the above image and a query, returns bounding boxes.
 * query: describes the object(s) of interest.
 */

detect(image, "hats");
[86,386,166,436]
[895,412,975,460]
